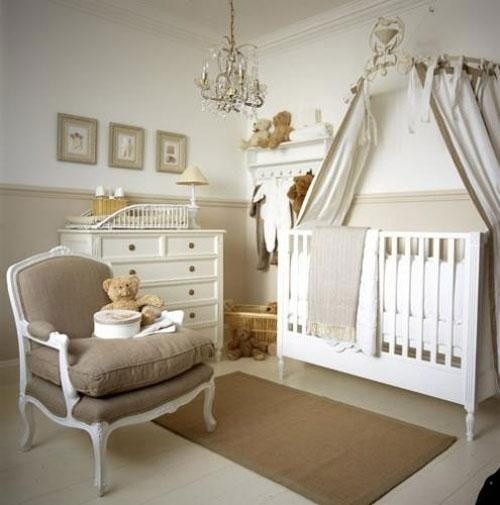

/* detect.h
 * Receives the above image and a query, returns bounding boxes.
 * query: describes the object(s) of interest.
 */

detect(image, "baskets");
[223,299,277,344]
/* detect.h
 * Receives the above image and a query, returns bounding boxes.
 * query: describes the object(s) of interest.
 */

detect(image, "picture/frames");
[56,112,188,176]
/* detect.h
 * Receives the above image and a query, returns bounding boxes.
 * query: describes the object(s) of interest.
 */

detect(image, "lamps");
[192,1,265,121]
[175,165,210,230]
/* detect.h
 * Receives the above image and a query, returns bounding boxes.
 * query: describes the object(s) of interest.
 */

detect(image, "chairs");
[6,244,219,495]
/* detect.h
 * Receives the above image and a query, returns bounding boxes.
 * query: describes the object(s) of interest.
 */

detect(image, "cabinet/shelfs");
[57,226,226,365]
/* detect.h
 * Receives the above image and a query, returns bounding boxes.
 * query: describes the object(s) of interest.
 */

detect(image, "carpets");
[151,369,458,504]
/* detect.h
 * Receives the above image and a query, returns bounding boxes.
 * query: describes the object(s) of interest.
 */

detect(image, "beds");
[275,216,498,443]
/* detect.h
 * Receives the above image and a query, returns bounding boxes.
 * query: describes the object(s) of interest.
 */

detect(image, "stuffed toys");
[100,274,163,324]
[228,326,268,360]
[266,303,277,315]
[241,110,294,150]
[287,175,313,213]
